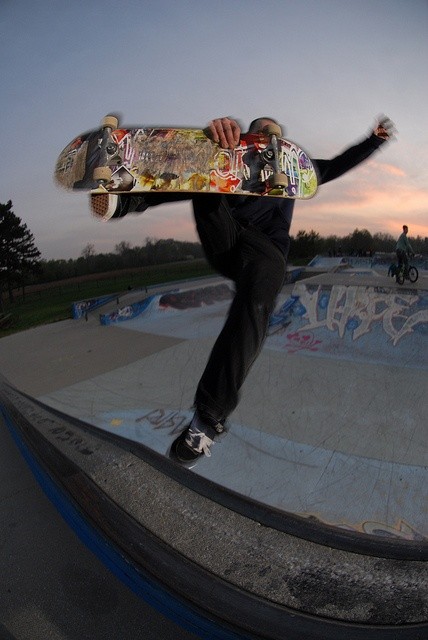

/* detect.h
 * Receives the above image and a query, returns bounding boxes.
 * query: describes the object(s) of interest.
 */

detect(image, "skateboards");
[54,115,318,200]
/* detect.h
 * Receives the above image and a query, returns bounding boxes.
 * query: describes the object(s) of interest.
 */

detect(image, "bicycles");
[388,253,418,285]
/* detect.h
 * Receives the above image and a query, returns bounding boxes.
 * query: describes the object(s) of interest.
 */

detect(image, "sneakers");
[166,416,231,470]
[88,194,142,223]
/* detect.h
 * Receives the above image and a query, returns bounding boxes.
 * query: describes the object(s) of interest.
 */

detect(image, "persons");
[388,263,398,277]
[395,225,415,283]
[328,244,376,258]
[88,115,397,468]
